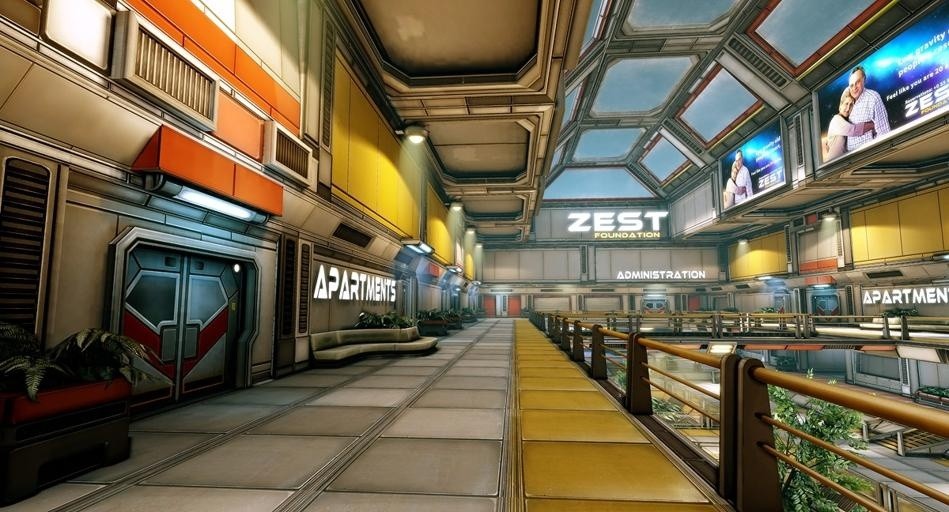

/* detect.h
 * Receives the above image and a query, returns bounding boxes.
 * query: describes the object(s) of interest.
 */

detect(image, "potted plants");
[0,321,177,424]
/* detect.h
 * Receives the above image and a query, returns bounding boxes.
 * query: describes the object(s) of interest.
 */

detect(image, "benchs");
[309,324,439,365]
